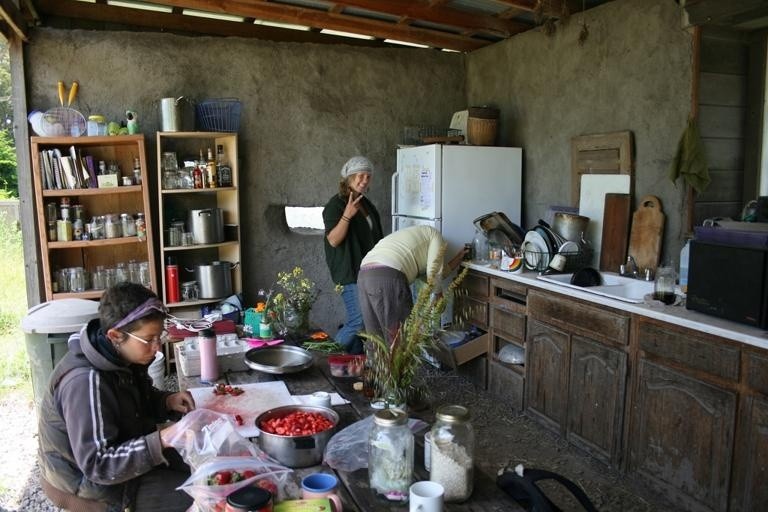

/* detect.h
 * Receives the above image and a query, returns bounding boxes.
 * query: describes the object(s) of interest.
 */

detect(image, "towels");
[667,117,718,195]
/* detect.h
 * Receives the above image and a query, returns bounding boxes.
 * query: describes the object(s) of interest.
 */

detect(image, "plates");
[521,219,581,271]
[717,221,768,233]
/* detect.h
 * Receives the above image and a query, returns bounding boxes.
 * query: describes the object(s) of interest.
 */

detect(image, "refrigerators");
[393,144,525,329]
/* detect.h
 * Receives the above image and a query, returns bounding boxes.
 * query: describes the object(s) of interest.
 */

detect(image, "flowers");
[275,264,346,311]
[257,275,285,324]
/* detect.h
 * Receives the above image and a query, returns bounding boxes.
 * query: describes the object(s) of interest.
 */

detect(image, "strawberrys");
[260,410,334,437]
[212,383,244,396]
[209,470,277,512]
[236,414,243,426]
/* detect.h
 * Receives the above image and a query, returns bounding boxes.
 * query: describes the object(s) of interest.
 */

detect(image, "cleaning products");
[679,239,693,285]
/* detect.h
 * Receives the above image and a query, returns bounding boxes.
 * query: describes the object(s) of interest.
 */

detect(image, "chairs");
[496,467,599,511]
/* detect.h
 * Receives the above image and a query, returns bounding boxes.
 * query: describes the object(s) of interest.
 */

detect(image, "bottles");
[165,220,192,246]
[92,260,150,289]
[164,256,180,303]
[197,328,219,381]
[95,160,118,174]
[679,239,691,293]
[133,158,142,184]
[120,174,131,186]
[182,281,197,302]
[192,145,232,187]
[224,487,274,512]
[46,198,147,242]
[464,243,471,260]
[51,267,85,292]
[162,153,194,189]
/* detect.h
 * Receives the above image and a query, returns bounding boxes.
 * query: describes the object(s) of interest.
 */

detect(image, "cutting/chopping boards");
[599,192,630,273]
[578,173,629,270]
[625,195,665,274]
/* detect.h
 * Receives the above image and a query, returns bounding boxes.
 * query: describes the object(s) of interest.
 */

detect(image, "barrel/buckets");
[147,351,166,391]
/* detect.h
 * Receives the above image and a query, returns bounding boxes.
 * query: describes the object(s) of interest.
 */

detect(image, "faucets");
[627,255,639,274]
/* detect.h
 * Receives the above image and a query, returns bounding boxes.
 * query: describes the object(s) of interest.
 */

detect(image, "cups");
[507,257,523,274]
[408,481,444,512]
[424,430,431,472]
[311,390,332,408]
[302,473,341,512]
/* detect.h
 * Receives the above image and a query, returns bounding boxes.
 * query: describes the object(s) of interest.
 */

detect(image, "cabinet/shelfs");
[156,129,244,378]
[433,273,488,389]
[28,132,159,303]
[526,284,630,473]
[630,317,744,512]
[743,344,768,512]
[488,276,526,412]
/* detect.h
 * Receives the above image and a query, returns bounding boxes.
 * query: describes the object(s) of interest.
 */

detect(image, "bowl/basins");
[496,343,523,365]
[200,306,240,325]
[326,353,366,378]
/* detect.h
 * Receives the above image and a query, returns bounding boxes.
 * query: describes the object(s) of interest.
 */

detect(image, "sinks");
[582,278,684,301]
[536,270,628,287]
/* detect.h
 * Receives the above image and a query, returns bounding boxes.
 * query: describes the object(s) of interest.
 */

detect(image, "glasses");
[121,328,171,352]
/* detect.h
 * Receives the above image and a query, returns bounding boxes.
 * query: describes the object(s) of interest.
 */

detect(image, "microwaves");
[684,239,768,331]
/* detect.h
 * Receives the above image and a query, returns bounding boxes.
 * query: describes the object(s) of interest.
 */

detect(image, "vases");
[282,306,308,340]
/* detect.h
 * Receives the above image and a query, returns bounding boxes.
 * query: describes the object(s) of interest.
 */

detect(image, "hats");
[339,156,373,178]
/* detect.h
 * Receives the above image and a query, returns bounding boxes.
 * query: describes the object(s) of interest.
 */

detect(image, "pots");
[553,211,591,245]
[152,96,196,135]
[189,208,226,244]
[254,404,340,467]
[183,260,239,299]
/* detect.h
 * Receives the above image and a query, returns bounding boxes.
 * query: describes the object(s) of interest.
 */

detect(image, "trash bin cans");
[21,298,101,424]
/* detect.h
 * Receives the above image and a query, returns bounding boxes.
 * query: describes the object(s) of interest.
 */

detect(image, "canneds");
[654,268,676,305]
[47,169,150,242]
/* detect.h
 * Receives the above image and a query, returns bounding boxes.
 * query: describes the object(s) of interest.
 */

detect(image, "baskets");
[467,116,496,145]
[196,97,240,131]
[469,104,499,119]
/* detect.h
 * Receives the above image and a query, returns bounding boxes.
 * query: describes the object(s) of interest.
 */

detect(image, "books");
[38,145,97,190]
[186,380,296,439]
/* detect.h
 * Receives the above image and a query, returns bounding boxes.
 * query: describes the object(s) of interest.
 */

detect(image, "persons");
[39,284,196,512]
[323,156,383,353]
